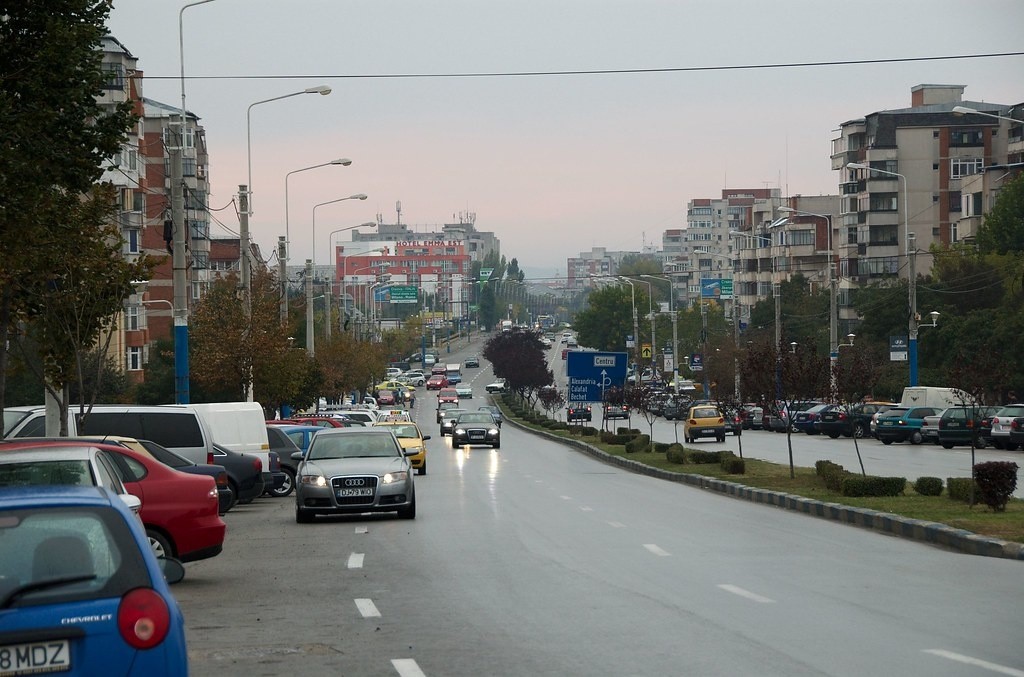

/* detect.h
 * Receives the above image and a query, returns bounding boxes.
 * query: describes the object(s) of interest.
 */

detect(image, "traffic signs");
[566,351,628,403]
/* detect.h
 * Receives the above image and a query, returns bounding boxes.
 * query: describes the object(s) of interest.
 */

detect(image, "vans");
[3,402,215,466]
[901,386,978,409]
[158,403,279,490]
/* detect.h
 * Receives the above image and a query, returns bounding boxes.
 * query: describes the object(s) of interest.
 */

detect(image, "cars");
[601,401,630,420]
[0,446,148,587]
[0,485,190,677]
[566,403,593,423]
[0,432,268,576]
[541,322,1024,454]
[289,426,419,524]
[719,410,742,436]
[682,406,726,443]
[256,349,506,498]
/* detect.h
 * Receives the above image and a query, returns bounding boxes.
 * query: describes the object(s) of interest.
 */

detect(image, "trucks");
[502,320,513,333]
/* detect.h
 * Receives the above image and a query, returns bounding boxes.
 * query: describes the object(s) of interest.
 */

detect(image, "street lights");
[238,84,400,358]
[592,248,743,405]
[728,230,798,400]
[778,205,855,404]
[845,162,940,384]
